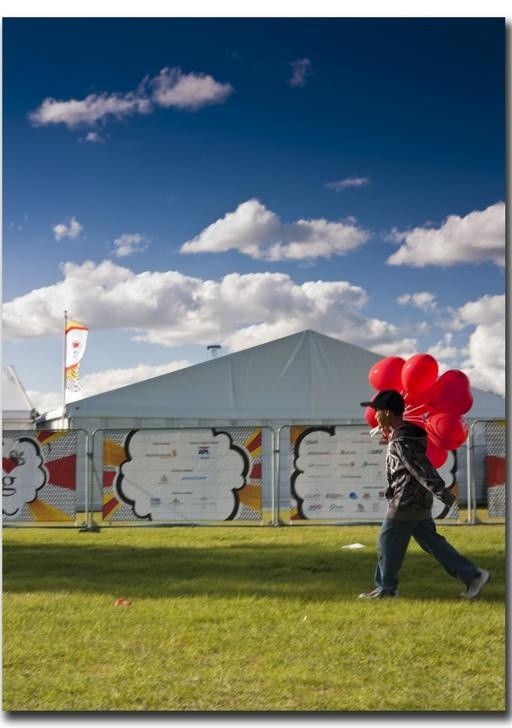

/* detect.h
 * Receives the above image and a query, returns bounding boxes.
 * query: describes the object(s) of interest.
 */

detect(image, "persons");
[357,390,493,602]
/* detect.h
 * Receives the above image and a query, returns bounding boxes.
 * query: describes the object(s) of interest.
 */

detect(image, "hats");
[361,390,404,412]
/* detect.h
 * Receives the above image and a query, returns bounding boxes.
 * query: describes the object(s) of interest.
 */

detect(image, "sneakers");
[358,589,398,599]
[460,567,490,597]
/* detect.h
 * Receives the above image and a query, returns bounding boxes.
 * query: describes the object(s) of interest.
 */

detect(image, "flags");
[64,319,89,390]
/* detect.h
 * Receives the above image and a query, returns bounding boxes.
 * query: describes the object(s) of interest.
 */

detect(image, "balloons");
[364,353,474,469]
